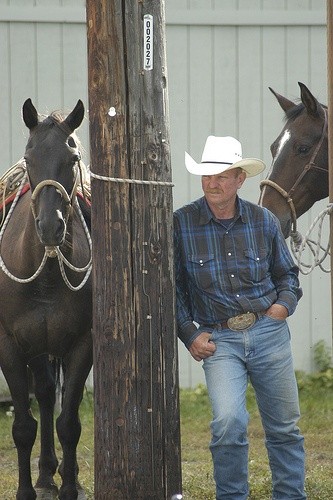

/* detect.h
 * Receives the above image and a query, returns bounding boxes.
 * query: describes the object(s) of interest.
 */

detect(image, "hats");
[185,135,266,178]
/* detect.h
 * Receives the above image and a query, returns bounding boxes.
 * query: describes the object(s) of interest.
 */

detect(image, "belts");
[200,311,257,331]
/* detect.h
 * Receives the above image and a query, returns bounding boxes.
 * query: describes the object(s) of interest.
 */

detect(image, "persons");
[173,135,307,500]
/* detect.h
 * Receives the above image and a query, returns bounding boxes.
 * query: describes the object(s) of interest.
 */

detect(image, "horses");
[1,98,94,500]
[253,82,328,240]
